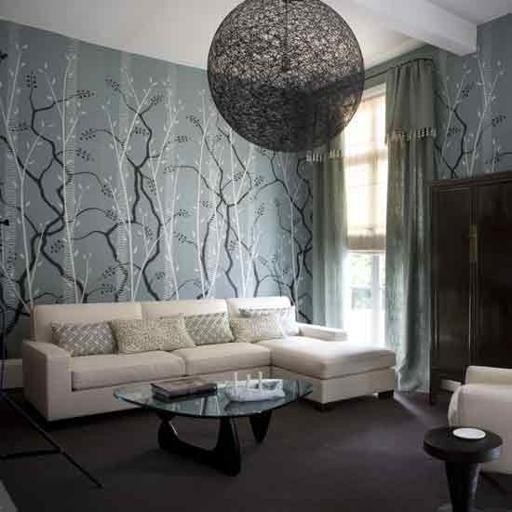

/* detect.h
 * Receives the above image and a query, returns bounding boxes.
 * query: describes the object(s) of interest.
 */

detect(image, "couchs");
[20,299,271,434]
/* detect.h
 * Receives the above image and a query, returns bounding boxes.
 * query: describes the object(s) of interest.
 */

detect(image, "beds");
[228,295,397,412]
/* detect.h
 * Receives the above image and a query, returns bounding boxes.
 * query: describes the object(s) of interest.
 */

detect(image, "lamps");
[207,0,365,152]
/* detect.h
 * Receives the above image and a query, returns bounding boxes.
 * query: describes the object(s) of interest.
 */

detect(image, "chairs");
[447,364,512,476]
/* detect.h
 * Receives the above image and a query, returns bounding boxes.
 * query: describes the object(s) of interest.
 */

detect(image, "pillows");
[50,305,300,358]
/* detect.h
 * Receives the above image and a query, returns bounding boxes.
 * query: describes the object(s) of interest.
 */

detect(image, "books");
[150,377,217,398]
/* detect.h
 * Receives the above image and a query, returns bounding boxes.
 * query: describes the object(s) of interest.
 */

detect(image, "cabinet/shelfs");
[428,171,511,405]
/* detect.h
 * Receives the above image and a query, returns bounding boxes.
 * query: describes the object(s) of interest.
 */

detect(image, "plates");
[453,427,485,439]
